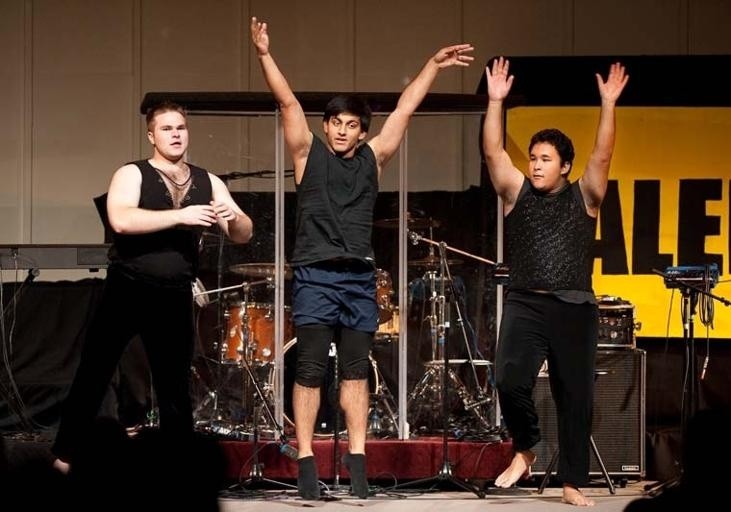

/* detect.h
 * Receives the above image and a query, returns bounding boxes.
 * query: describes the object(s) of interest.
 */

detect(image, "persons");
[247,15,476,500]
[482,56,630,508]
[55,100,255,461]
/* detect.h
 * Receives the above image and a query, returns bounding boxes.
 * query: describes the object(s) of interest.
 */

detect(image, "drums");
[374,307,399,342]
[268,336,381,438]
[376,268,395,325]
[221,300,294,366]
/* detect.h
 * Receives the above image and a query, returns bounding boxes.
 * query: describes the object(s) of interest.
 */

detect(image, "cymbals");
[409,257,463,267]
[201,232,233,249]
[229,263,292,280]
[374,214,440,230]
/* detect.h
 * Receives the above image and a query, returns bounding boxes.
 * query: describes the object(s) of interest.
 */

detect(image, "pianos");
[0,244,111,269]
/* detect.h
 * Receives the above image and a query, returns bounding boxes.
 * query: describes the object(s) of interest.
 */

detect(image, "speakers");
[527,346,646,477]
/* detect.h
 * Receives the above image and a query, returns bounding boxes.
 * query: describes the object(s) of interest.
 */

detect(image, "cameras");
[662,263,719,291]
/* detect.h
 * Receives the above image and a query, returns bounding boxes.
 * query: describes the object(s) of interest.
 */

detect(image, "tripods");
[193,279,288,440]
[1,269,43,440]
[645,296,720,495]
[215,290,305,500]
[537,426,617,495]
[386,246,503,437]
[369,257,508,501]
